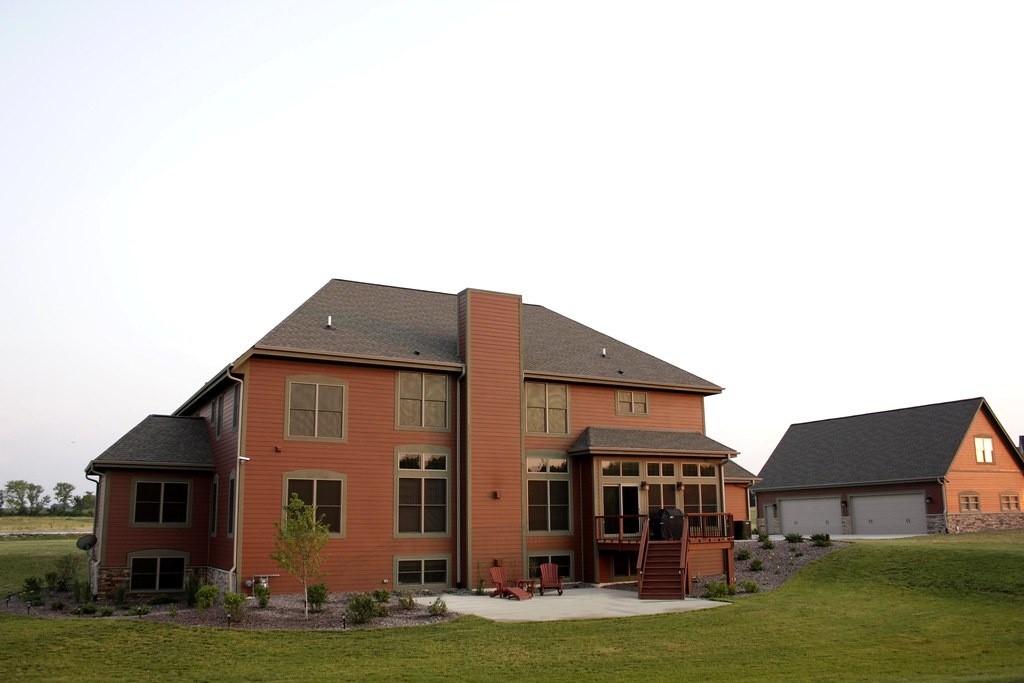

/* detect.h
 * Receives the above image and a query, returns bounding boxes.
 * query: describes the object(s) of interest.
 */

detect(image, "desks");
[518,580,535,597]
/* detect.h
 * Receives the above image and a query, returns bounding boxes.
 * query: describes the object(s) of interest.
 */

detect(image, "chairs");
[489,567,530,601]
[539,563,564,596]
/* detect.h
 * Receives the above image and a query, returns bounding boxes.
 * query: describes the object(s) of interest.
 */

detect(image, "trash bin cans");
[734,521,752,540]
